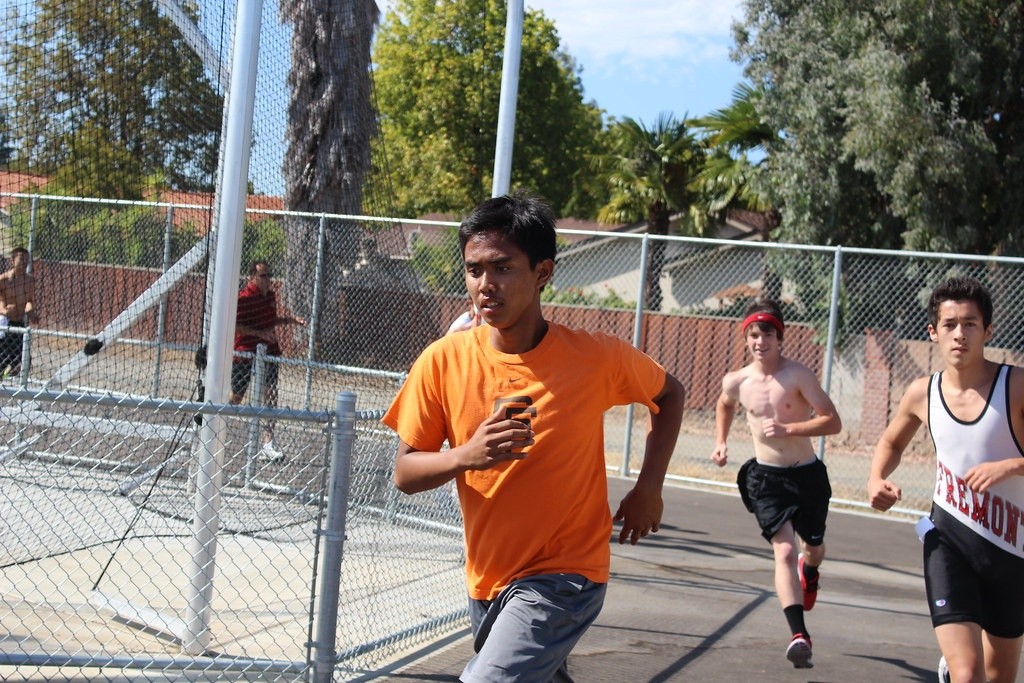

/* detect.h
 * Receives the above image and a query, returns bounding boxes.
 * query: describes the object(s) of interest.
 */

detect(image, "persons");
[224,260,308,462]
[0,247,36,384]
[0,254,40,325]
[382,192,687,683]
[867,276,1024,683]
[445,293,486,335]
[709,302,843,669]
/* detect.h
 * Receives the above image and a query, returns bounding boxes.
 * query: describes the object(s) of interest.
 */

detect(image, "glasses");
[255,272,274,279]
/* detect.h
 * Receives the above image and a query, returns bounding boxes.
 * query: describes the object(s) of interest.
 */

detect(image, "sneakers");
[797,553,820,612]
[259,440,285,463]
[786,634,815,670]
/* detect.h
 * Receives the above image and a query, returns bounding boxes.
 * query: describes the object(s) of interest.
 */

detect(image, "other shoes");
[938,657,950,683]
